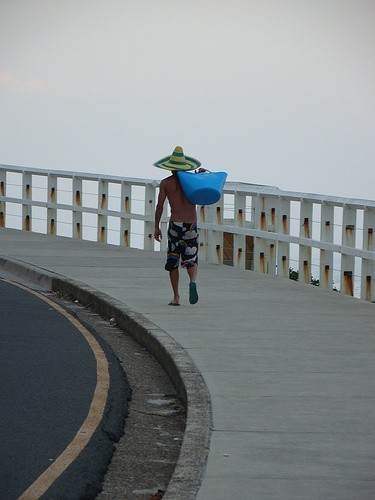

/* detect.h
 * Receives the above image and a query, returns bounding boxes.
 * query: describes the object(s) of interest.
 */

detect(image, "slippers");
[168,301,180,305]
[189,281,199,305]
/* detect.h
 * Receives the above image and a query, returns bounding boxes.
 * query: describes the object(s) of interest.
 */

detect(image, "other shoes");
[153,146,202,171]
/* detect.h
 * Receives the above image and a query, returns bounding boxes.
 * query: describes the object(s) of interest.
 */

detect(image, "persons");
[154,146,206,306]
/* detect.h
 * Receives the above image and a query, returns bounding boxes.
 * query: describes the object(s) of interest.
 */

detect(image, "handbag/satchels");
[179,171,228,206]
